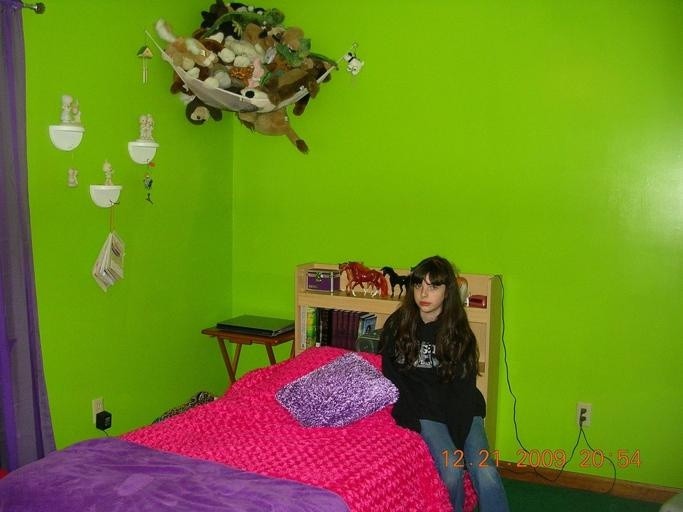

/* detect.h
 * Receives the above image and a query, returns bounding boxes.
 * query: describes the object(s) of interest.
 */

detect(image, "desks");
[201,326,294,386]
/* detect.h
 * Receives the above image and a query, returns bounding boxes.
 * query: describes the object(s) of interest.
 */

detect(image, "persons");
[380,256,510,510]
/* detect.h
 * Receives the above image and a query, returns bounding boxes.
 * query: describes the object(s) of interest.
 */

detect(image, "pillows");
[275,351,401,428]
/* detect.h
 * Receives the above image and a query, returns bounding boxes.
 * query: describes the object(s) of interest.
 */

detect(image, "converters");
[96,410,112,430]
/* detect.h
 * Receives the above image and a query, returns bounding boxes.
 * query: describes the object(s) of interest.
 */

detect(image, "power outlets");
[91,397,104,425]
[575,403,591,427]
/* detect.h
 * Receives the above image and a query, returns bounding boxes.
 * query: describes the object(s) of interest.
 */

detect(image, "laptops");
[216,313,295,339]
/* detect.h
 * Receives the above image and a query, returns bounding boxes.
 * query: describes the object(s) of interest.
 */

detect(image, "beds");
[0,348,502,510]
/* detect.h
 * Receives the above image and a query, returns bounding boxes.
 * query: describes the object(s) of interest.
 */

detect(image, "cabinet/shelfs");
[292,262,502,460]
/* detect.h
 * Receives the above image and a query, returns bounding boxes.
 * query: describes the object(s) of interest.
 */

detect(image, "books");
[298,303,377,352]
[92,231,127,297]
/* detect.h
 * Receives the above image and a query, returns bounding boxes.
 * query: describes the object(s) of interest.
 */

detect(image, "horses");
[339,262,375,296]
[344,261,389,298]
[455,277,468,306]
[380,267,408,299]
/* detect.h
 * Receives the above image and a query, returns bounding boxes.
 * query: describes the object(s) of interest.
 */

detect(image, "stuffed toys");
[155,0,339,157]
